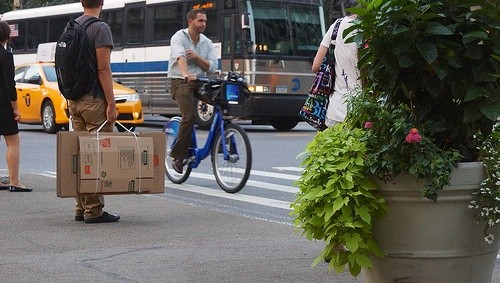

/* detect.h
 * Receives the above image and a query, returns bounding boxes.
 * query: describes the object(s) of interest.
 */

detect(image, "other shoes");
[0,184,10,190]
[85,211,120,223]
[9,186,33,192]
[172,158,183,173]
[75,216,84,221]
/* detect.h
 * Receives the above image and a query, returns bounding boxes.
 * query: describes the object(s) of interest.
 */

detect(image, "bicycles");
[162,70,253,194]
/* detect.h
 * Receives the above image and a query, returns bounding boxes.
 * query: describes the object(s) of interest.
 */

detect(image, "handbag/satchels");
[298,17,343,133]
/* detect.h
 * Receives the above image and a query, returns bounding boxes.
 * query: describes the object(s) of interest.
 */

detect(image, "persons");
[168,9,230,174]
[63,0,120,223]
[312,11,368,262]
[0,21,34,192]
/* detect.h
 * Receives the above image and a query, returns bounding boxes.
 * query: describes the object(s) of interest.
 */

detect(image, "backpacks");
[55,17,103,100]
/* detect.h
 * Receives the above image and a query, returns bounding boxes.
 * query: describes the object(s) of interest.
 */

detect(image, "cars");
[16,62,144,133]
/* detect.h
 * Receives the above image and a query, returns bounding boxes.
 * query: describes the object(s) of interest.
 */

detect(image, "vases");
[362,161,497,283]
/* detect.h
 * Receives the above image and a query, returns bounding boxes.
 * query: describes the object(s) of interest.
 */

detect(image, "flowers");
[294,0,500,283]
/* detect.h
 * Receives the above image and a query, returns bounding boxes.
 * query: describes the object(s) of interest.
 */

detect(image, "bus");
[0,0,327,132]
